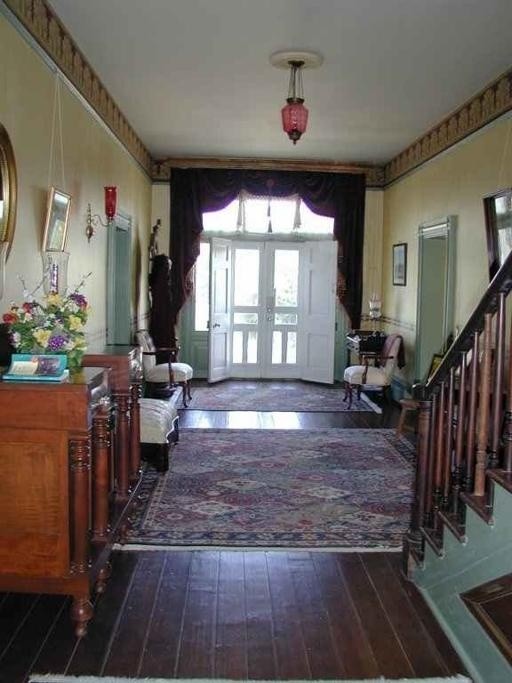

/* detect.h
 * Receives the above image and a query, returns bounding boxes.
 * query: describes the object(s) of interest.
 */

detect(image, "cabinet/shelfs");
[1,367,110,637]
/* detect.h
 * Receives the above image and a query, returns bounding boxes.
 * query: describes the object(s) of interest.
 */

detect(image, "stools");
[394,399,424,438]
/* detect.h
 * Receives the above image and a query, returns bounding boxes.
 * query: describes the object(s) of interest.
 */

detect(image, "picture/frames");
[41,185,72,251]
[480,186,512,282]
[392,243,406,286]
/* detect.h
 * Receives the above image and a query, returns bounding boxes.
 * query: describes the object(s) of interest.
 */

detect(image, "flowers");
[3,272,94,372]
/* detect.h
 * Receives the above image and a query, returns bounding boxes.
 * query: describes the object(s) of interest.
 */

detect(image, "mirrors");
[1,121,17,259]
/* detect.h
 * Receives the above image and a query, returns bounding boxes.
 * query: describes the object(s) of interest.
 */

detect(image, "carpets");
[174,386,381,414]
[109,427,418,551]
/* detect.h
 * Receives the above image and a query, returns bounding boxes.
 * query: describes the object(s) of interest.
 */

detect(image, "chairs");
[129,329,192,471]
[342,335,405,409]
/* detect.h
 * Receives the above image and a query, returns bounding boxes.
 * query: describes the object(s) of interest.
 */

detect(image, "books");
[2,353,70,382]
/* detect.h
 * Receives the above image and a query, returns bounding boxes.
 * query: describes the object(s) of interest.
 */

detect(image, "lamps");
[85,186,117,241]
[270,50,324,145]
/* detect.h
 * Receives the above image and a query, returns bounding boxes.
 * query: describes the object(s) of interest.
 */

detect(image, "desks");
[344,332,387,399]
